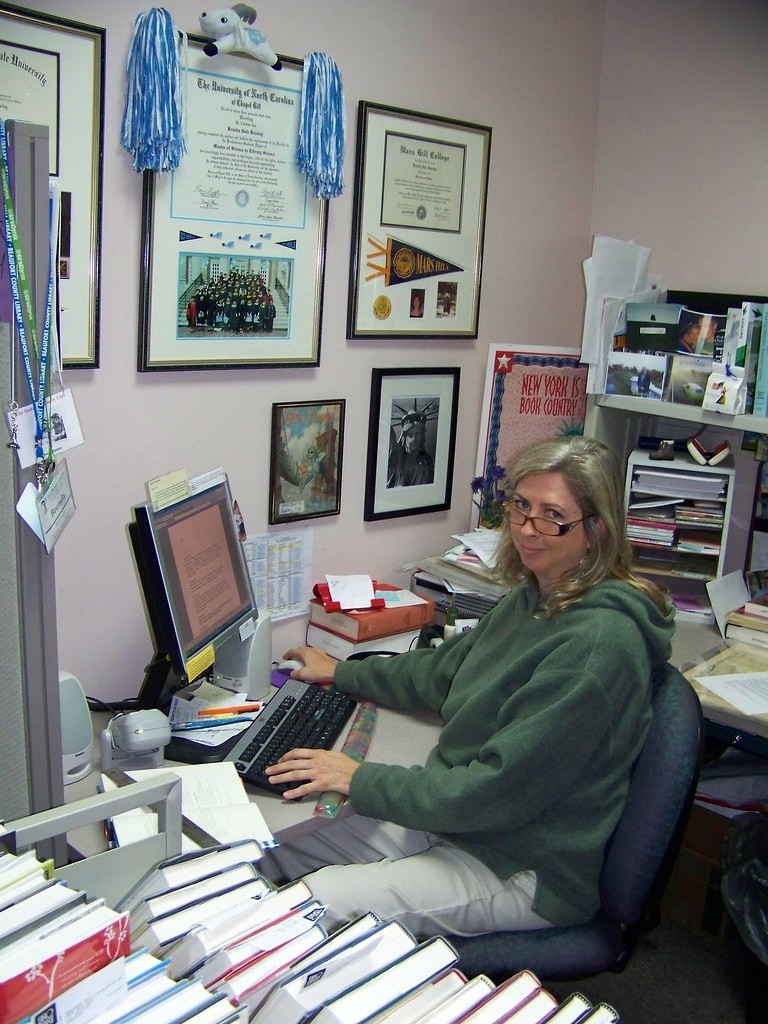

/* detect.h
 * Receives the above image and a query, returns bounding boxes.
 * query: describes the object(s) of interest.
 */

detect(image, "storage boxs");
[658,804,733,945]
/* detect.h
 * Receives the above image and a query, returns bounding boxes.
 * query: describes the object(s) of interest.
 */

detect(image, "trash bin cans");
[745,857,768,1024]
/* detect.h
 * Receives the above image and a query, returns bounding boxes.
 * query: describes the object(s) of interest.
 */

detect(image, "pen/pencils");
[172,716,252,732]
[198,704,260,715]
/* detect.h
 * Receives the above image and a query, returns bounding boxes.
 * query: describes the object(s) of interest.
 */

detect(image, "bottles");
[444,607,459,642]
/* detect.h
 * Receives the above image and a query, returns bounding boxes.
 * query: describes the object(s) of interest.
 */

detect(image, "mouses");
[277,657,317,685]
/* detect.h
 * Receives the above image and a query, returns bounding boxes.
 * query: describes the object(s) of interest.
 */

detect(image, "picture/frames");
[268,399,346,526]
[346,100,492,340]
[0,0,106,370]
[469,343,590,532]
[137,32,330,372]
[363,367,461,522]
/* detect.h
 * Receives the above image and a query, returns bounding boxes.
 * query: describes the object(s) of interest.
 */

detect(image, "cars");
[682,384,704,400]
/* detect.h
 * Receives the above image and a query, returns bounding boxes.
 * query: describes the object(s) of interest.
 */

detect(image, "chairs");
[444,659,705,987]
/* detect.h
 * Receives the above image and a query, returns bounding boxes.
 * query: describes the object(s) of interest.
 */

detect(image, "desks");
[90,686,447,853]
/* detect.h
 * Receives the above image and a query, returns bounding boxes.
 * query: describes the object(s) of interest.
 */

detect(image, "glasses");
[502,498,599,537]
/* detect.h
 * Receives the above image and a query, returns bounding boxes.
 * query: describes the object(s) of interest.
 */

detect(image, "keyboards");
[223,679,358,802]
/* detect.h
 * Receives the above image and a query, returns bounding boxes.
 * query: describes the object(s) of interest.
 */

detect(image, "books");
[721,301,768,418]
[0,833,620,1024]
[415,536,515,620]
[307,583,434,663]
[625,469,728,577]
[723,592,768,649]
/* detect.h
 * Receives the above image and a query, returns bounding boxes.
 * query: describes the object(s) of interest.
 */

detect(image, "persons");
[184,267,276,334]
[636,366,650,397]
[679,316,718,356]
[233,501,247,539]
[256,434,674,940]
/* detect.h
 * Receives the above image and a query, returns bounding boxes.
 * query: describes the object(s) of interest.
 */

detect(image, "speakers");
[59,670,94,786]
[212,607,271,702]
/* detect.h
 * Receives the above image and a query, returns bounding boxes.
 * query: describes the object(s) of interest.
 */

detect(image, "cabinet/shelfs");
[412,288,768,737]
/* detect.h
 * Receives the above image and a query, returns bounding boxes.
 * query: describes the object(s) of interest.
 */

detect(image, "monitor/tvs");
[127,466,253,764]
